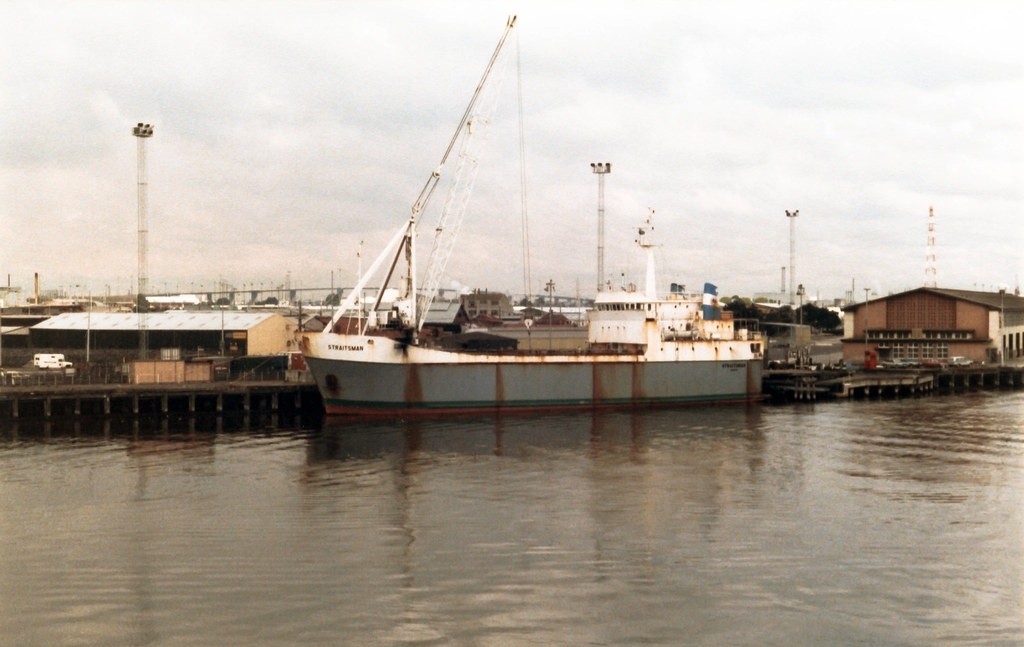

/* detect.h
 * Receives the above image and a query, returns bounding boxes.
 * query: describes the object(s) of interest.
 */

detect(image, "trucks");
[34,353,73,369]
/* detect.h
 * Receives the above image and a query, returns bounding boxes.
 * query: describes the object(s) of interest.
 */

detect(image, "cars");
[1,370,30,380]
[766,355,973,372]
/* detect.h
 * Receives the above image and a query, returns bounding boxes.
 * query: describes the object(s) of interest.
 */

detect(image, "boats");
[294,13,765,415]
